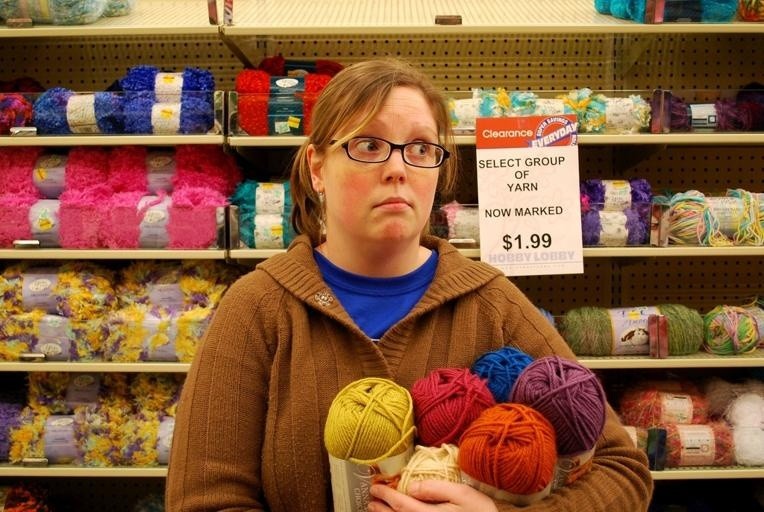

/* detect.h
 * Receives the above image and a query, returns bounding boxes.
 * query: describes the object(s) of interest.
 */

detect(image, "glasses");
[330,136,451,167]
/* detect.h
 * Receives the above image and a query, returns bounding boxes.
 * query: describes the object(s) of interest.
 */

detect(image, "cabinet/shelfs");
[0,0,764,512]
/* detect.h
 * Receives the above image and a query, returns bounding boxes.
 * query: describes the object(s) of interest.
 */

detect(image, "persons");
[160,55,658,511]
